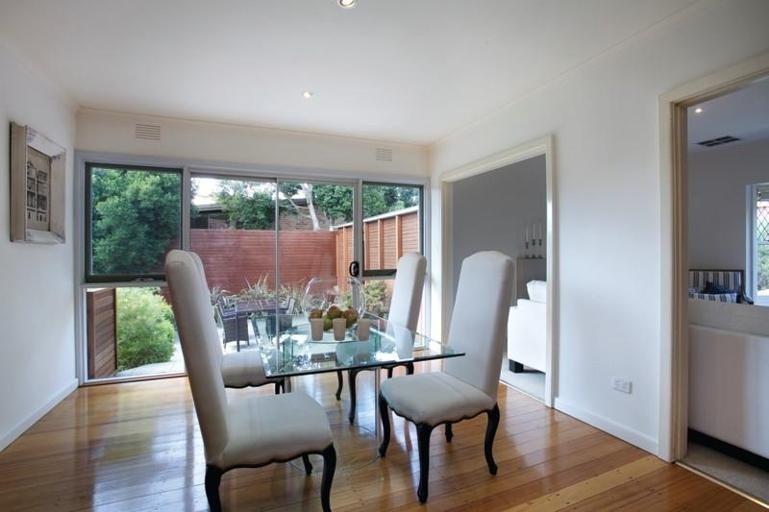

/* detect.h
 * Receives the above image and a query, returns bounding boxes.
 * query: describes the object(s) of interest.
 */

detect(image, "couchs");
[508,278,768,468]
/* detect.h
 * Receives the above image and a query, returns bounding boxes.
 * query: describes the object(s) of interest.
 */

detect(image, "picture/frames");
[6,120,67,248]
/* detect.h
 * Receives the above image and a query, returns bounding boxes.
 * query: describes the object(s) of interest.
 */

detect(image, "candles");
[524,221,543,243]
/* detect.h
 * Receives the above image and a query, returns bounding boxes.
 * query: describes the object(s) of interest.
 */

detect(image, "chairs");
[166,249,515,512]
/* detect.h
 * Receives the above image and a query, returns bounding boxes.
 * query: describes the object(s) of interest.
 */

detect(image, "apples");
[308,305,359,332]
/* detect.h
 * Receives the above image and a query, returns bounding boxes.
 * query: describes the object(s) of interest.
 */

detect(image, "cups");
[310,318,323,341]
[357,318,370,340]
[333,318,347,340]
